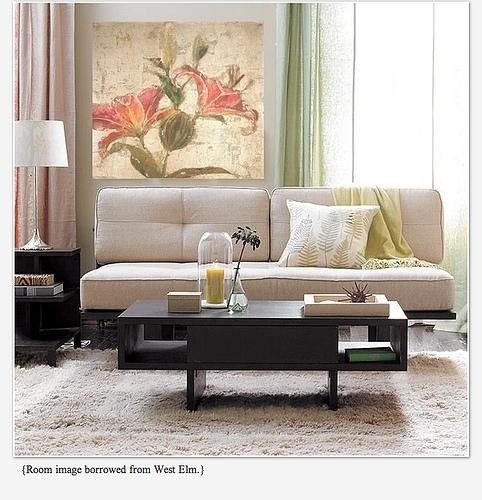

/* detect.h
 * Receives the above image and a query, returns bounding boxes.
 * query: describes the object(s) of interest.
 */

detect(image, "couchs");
[79,184,456,340]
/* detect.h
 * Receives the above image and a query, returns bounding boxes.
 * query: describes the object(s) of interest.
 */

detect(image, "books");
[14,272,63,296]
[344,347,396,362]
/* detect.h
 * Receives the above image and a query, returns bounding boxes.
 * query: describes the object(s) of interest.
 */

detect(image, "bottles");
[229,267,248,312]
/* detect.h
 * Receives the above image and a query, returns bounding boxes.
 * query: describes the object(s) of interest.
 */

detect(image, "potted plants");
[227,223,261,312]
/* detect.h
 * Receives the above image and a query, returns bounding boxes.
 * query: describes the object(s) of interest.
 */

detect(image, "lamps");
[11,119,70,252]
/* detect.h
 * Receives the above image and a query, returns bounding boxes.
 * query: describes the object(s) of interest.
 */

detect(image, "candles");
[204,267,226,304]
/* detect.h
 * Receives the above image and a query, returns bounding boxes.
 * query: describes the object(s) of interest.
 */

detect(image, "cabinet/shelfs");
[14,247,82,366]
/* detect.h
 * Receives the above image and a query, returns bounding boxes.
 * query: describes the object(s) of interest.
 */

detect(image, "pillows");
[277,196,378,267]
